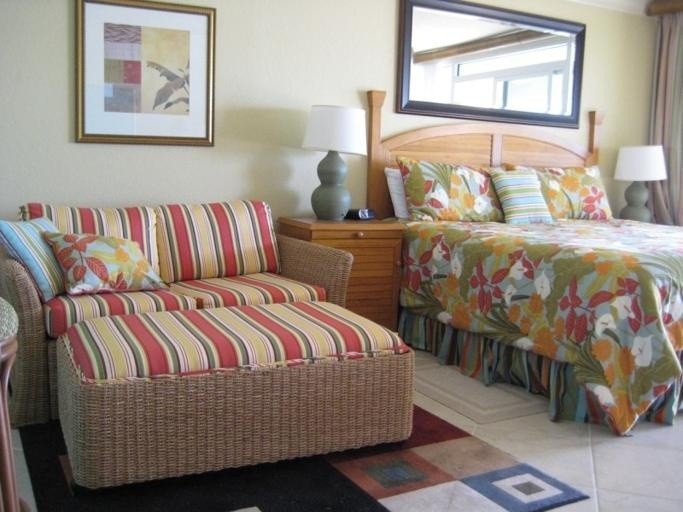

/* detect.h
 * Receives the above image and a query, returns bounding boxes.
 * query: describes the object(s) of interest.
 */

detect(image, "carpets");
[16,409,590,512]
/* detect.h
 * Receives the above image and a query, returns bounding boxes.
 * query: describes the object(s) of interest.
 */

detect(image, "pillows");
[380,153,613,225]
[1,214,169,300]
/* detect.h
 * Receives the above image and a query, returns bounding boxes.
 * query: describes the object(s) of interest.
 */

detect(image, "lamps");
[299,101,370,220]
[613,144,669,222]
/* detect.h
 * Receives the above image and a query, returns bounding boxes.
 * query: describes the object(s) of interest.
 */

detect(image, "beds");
[368,90,683,423]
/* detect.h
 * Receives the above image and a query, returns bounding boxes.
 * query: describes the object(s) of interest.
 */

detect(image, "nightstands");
[277,214,406,335]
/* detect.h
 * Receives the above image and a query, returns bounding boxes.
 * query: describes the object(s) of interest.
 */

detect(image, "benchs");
[52,299,417,492]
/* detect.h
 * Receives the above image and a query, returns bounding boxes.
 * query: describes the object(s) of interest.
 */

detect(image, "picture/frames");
[75,1,216,146]
[396,0,585,129]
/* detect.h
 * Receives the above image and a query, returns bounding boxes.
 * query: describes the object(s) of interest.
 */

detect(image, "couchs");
[2,201,355,427]
